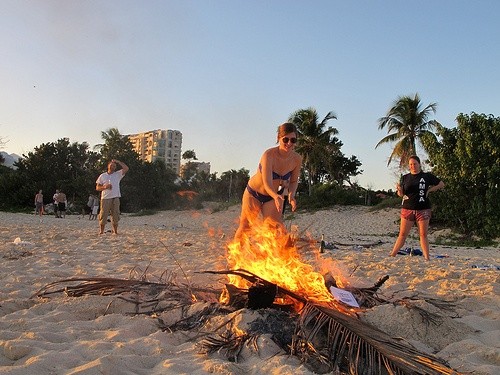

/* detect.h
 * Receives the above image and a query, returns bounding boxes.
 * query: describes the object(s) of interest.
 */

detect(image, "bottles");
[400,174,403,187]
[109,178,112,190]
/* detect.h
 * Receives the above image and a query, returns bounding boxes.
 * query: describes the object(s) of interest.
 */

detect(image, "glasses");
[282,137,297,143]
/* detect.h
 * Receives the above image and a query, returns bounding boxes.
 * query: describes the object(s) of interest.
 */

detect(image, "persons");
[389,156,446,260]
[35,159,130,235]
[233,123,303,242]
